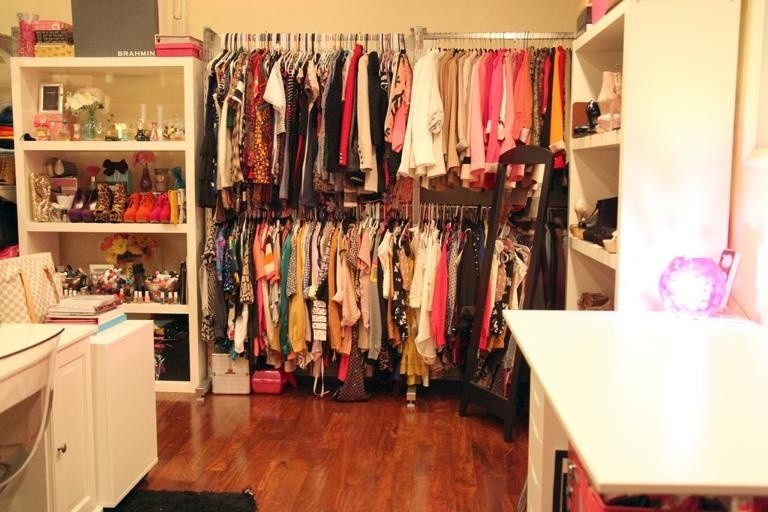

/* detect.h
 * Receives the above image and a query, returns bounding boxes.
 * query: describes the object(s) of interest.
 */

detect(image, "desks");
[499,309,768,511]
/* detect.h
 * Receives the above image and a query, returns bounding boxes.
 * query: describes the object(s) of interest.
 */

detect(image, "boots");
[95,183,171,224]
[570,196,617,254]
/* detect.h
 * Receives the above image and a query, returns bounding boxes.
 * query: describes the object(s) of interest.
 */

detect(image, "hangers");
[428,31,575,52]
[216,204,489,234]
[205,32,390,75]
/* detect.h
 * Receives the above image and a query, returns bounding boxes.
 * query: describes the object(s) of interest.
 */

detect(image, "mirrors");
[459,144,555,443]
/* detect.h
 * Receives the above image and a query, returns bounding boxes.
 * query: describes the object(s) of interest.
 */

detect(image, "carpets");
[102,487,256,512]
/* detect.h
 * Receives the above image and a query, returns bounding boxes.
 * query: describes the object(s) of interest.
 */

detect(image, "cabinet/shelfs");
[11,56,210,393]
[567,0,742,309]
[47,336,97,512]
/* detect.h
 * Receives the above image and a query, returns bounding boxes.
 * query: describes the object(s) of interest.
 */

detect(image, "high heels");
[585,99,601,131]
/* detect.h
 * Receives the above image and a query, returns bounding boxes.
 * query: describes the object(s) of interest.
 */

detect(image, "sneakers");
[579,292,614,310]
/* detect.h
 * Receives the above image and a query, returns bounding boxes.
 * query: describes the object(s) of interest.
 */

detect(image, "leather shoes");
[81,188,97,222]
[67,187,89,221]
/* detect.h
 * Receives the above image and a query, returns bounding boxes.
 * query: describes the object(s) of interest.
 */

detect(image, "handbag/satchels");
[0,252,63,324]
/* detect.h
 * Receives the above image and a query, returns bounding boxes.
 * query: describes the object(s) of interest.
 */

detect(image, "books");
[42,293,126,326]
[155,33,202,47]
